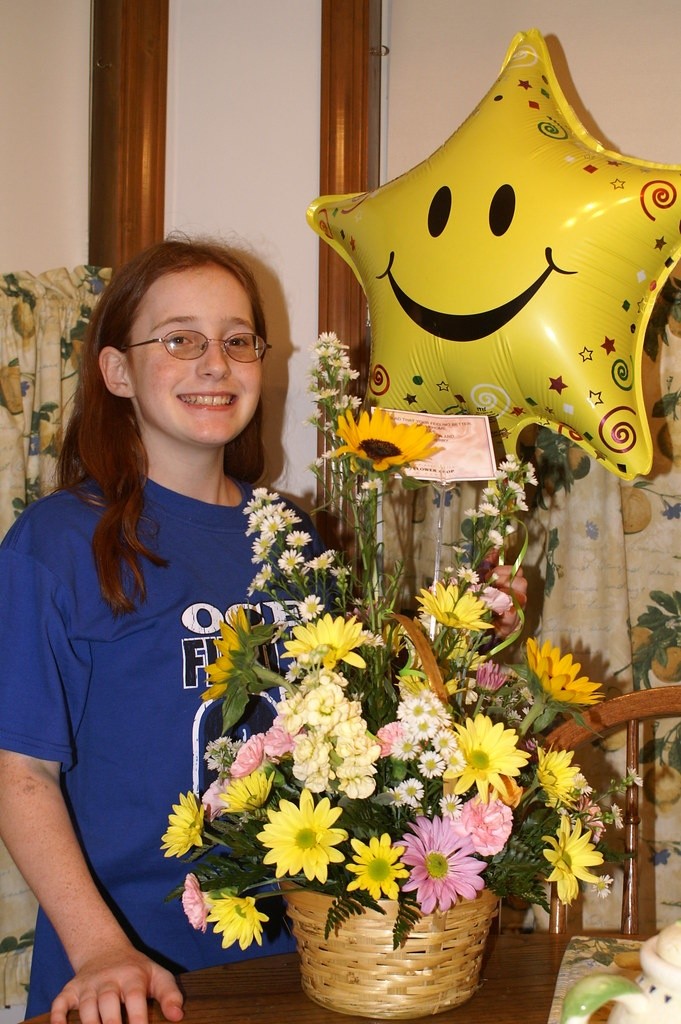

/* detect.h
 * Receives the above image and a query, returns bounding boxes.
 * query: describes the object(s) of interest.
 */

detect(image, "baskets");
[277,607,500,1020]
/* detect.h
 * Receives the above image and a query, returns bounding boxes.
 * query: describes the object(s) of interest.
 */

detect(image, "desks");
[14,932,681,1024]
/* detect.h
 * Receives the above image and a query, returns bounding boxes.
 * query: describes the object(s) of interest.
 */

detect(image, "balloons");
[308,31,681,486]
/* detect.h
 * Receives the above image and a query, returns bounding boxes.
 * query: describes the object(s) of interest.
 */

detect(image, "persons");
[1,244,528,1024]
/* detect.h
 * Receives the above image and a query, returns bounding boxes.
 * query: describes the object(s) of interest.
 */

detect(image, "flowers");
[163,328,603,954]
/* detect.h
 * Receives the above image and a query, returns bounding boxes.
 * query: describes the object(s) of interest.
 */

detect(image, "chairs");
[495,685,681,941]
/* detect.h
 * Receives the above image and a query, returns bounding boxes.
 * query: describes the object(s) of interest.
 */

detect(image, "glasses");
[125,329,273,364]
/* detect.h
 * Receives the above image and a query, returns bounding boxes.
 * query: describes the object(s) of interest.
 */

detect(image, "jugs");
[561,920,681,1024]
